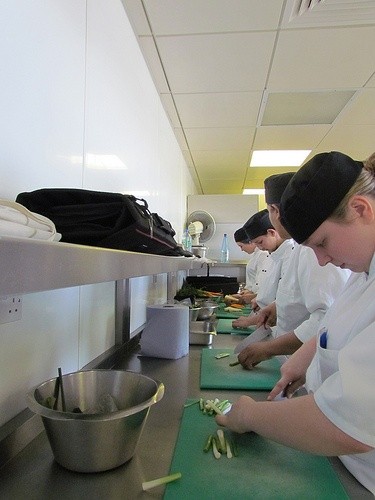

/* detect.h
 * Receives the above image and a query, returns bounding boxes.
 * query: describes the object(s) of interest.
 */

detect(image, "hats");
[279,151,363,244]
[234,228,249,242]
[243,209,272,240]
[264,172,296,203]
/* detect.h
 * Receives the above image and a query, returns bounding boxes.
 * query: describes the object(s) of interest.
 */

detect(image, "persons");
[233,228,269,304]
[237,171,351,370]
[216,151,375,494]
[232,209,295,328]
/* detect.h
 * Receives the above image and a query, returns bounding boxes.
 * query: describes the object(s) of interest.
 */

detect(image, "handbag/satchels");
[15,188,194,257]
[0,198,62,242]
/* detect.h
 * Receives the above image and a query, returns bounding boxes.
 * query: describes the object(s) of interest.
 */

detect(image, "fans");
[186,210,215,246]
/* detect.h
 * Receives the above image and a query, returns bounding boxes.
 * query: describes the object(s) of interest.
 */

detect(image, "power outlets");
[0,295,24,324]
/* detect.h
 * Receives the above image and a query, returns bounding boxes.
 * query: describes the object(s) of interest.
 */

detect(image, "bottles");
[182,229,192,253]
[221,234,229,263]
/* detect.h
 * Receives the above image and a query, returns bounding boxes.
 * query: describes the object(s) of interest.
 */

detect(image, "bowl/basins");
[25,369,166,473]
[188,295,222,322]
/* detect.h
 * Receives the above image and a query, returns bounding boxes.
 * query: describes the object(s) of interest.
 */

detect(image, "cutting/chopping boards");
[163,398,351,500]
[213,300,259,334]
[199,347,282,390]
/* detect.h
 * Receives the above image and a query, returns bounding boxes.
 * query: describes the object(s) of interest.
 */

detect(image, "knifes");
[233,323,273,357]
[248,309,257,318]
[266,381,290,401]
